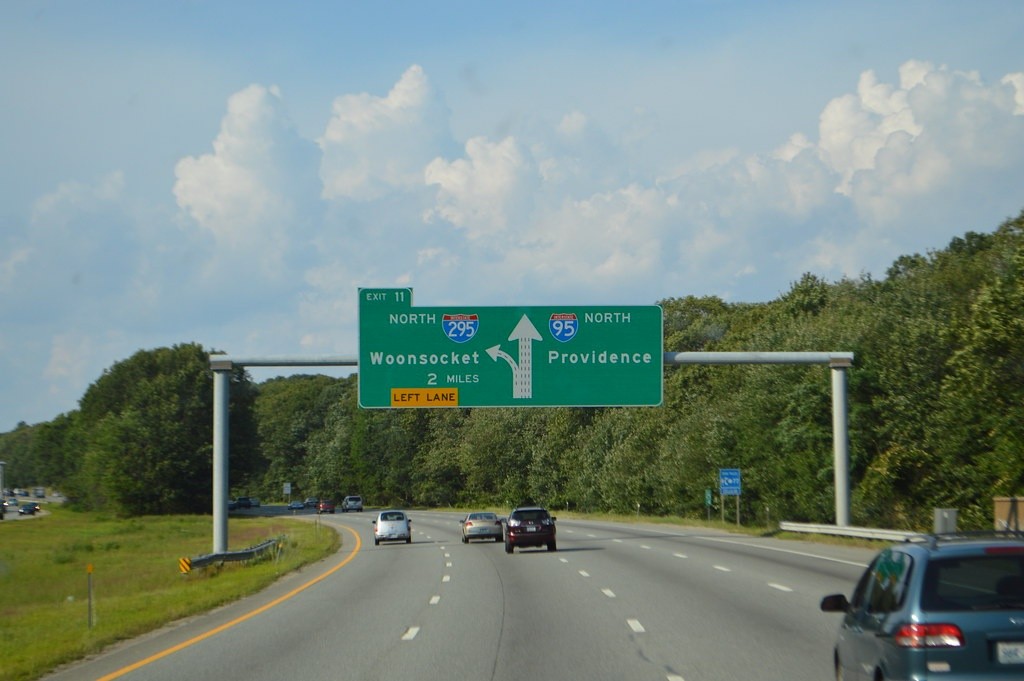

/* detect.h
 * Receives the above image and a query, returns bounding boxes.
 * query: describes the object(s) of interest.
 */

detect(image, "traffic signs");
[357,286,664,409]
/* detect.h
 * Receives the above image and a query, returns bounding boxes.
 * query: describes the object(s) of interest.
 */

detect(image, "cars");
[287,497,318,511]
[372,510,412,546]
[341,496,363,512]
[0,498,18,520]
[459,512,503,544]
[2,487,29,496]
[228,497,260,510]
[32,488,45,497]
[18,502,41,516]
[316,500,335,514]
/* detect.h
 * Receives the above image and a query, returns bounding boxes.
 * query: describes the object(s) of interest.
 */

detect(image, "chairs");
[990,574,1024,610]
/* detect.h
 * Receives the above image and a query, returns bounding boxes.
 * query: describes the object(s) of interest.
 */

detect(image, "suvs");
[501,505,556,554]
[820,530,1024,681]
